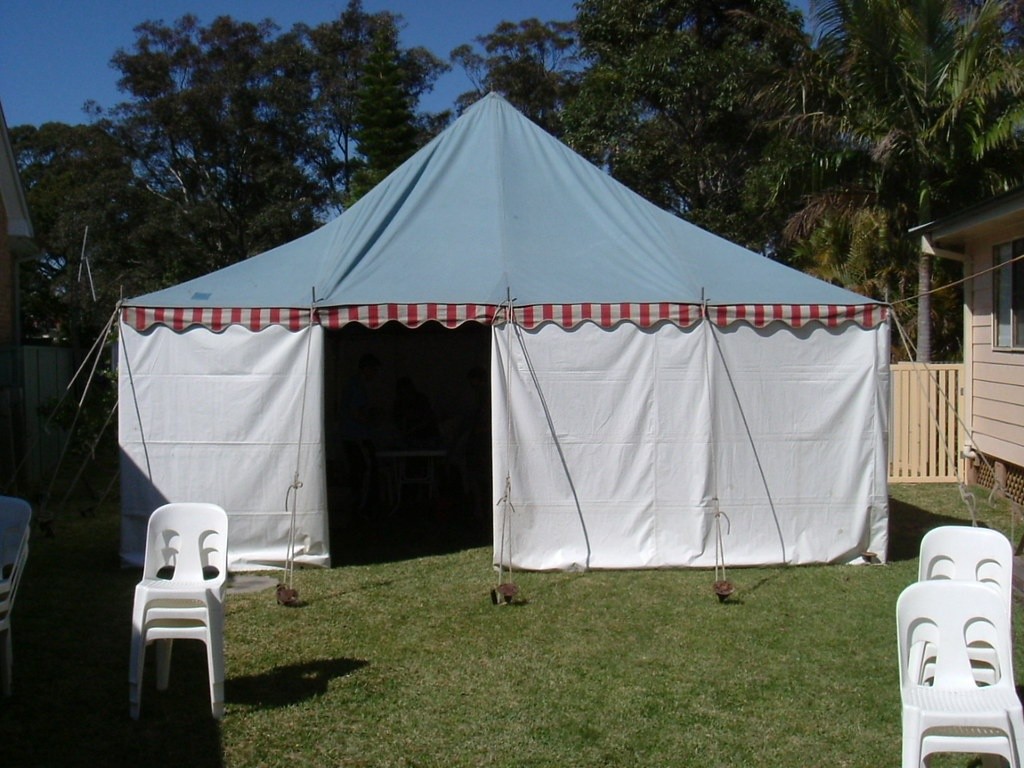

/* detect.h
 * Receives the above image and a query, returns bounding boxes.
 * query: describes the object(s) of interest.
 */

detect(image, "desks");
[376,450,457,519]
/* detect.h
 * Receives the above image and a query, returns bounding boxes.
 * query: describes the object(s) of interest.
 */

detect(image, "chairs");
[895,525,1024,768]
[0,497,31,697]
[128,501,230,720]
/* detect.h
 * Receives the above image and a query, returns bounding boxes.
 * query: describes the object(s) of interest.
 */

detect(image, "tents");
[117,93,894,571]
